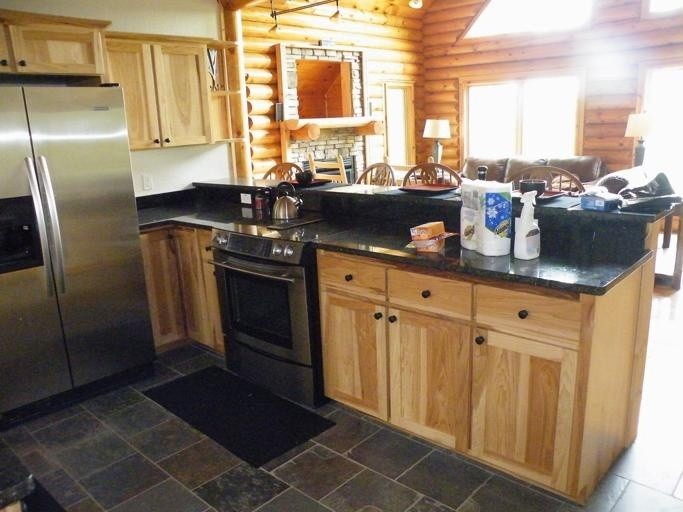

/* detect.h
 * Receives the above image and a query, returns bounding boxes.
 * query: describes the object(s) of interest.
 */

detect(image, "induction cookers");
[210,212,353,264]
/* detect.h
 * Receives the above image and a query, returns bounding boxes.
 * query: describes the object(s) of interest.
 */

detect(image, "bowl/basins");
[294,170,313,183]
[519,178,548,197]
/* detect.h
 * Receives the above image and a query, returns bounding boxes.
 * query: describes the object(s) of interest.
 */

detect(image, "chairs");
[505,166,585,196]
[261,153,461,192]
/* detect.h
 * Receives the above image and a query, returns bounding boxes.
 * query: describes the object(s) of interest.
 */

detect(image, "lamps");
[624,113,653,168]
[422,117,452,165]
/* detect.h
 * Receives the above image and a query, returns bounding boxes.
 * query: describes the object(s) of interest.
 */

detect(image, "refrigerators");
[0,78,158,434]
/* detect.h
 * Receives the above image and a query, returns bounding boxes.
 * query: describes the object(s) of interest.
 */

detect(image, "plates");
[510,188,569,203]
[398,180,459,195]
[286,177,332,187]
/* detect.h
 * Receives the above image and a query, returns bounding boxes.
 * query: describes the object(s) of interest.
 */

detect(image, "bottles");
[255,187,271,208]
[475,164,490,182]
[255,210,271,234]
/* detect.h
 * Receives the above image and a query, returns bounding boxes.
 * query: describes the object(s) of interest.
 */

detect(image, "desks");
[567,202,683,292]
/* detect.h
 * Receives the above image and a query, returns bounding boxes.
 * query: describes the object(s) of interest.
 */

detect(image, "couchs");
[457,155,601,187]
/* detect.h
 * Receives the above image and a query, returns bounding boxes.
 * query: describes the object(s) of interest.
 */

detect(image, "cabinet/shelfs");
[0,5,244,153]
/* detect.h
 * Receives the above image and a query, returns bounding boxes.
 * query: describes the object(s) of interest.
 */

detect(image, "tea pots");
[270,180,303,220]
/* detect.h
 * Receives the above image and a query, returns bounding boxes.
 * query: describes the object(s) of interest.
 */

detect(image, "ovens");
[207,249,326,408]
